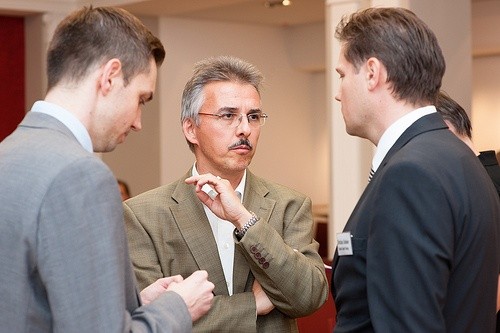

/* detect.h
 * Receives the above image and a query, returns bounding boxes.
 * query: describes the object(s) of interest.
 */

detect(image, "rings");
[216,176,222,180]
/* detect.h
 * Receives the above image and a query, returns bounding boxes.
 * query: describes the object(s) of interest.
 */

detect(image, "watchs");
[235,211,261,239]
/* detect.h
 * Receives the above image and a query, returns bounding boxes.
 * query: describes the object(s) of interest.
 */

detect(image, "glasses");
[193,107,268,129]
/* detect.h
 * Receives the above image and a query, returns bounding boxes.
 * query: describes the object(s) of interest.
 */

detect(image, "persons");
[330,8,500,333]
[117,180,130,201]
[121,57,329,333]
[0,6,214,333]
